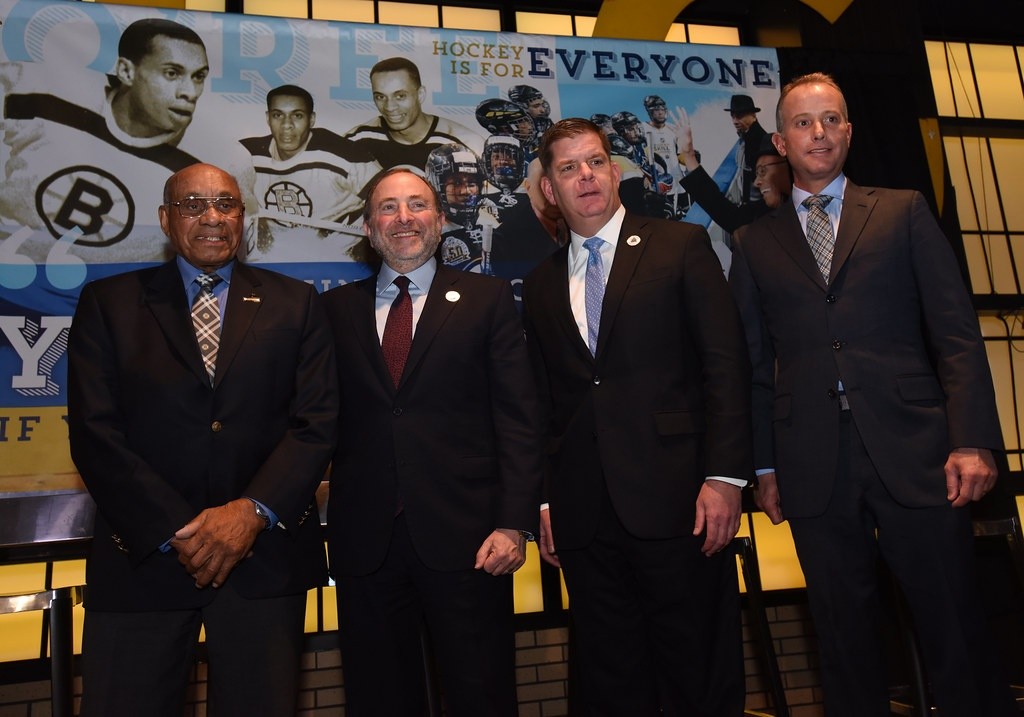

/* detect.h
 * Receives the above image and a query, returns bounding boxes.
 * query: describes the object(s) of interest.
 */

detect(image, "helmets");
[475,98,535,147]
[425,144,483,225]
[644,95,668,116]
[611,112,642,136]
[590,114,613,131]
[482,133,524,189]
[509,85,551,118]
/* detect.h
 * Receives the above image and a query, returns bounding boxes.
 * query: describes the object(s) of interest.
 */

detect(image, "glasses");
[753,161,787,178]
[167,195,245,218]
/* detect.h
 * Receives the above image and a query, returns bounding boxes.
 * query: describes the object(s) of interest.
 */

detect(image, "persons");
[519,115,754,717]
[320,167,547,717]
[65,159,345,717]
[3,16,790,272]
[727,71,1024,717]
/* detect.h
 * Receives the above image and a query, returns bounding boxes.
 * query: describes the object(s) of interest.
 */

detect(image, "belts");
[838,394,850,413]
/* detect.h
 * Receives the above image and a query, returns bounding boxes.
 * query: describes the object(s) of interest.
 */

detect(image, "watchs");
[256,502,271,528]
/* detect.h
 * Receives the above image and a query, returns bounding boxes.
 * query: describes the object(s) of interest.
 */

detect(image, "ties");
[801,195,835,286]
[191,272,221,390]
[582,237,606,360]
[382,276,412,391]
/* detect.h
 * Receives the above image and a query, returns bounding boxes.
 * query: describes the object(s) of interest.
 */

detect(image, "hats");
[724,94,761,112]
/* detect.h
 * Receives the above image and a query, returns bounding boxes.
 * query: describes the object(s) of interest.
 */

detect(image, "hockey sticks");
[480,205,493,276]
[672,154,692,215]
[258,208,364,237]
[646,131,660,195]
